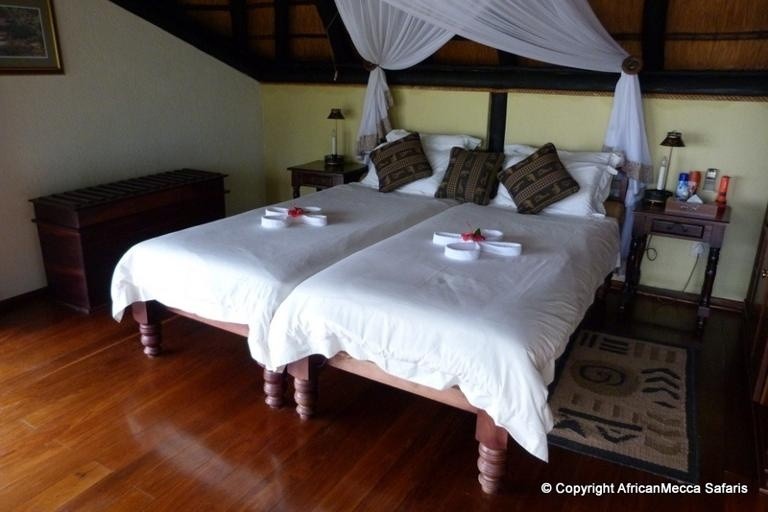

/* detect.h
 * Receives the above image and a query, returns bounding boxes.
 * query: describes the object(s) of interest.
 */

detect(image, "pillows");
[497,141,584,215]
[434,139,506,207]
[363,130,434,193]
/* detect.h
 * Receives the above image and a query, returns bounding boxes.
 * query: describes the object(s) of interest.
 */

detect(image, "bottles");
[676,166,730,220]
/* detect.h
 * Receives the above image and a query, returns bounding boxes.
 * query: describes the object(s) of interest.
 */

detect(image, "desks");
[285,159,369,200]
[26,166,231,319]
[614,189,737,330]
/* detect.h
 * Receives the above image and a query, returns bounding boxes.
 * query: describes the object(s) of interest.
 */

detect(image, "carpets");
[535,317,702,487]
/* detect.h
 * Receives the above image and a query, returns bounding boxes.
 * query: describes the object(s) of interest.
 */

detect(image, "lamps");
[643,127,686,203]
[324,107,345,164]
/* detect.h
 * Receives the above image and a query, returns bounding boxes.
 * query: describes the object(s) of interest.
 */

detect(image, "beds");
[106,127,486,414]
[262,140,646,499]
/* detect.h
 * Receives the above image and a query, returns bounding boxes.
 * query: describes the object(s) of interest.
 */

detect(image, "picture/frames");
[0,0,66,77]
